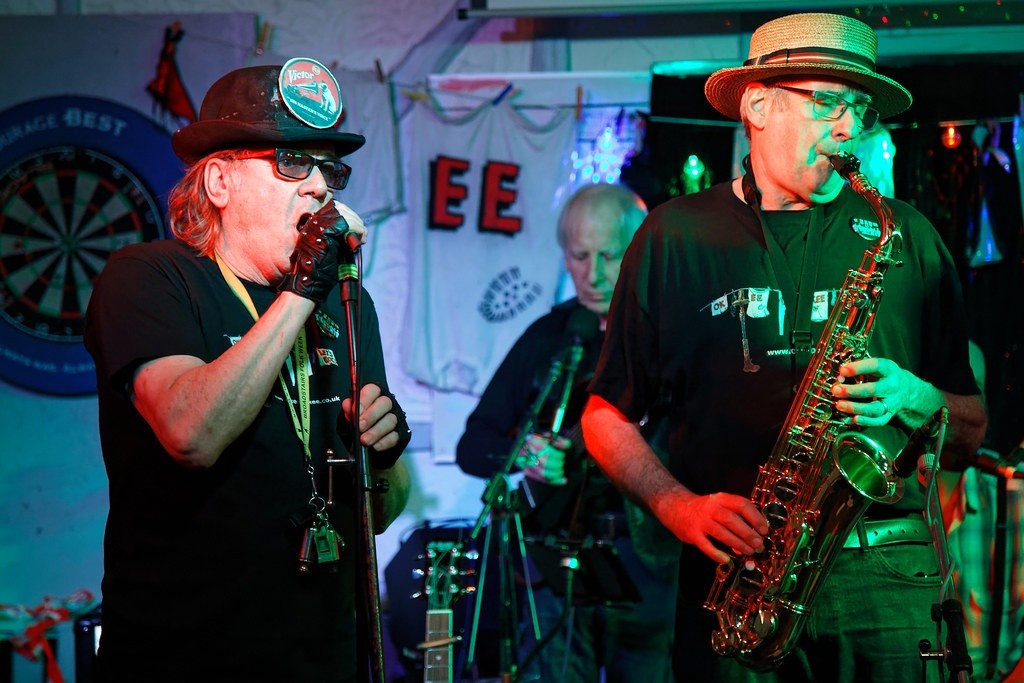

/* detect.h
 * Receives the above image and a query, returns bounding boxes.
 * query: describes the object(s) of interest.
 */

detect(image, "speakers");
[385,526,506,683]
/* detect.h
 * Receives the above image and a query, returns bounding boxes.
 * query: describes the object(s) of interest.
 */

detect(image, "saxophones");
[705,150,945,667]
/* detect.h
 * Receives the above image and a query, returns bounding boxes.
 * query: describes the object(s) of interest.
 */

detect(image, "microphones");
[892,407,948,480]
[343,229,362,251]
[549,309,599,437]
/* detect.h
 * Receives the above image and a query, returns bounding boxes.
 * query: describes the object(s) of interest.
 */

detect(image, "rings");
[879,399,888,415]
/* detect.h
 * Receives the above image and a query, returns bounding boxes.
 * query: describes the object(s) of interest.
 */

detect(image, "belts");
[840,518,932,548]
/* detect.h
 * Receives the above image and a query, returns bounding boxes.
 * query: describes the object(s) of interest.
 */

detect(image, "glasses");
[234,147,352,190]
[766,84,879,131]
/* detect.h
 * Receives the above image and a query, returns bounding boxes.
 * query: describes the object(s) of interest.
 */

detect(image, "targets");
[0,92,189,395]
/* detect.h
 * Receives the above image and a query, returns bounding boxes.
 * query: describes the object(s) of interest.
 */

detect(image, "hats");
[704,12,912,122]
[172,57,365,166]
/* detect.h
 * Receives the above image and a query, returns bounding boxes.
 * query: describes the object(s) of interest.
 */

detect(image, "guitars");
[412,536,482,683]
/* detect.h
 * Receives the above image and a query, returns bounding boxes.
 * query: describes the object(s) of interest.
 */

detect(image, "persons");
[578,14,988,683]
[455,181,687,683]
[80,56,413,683]
